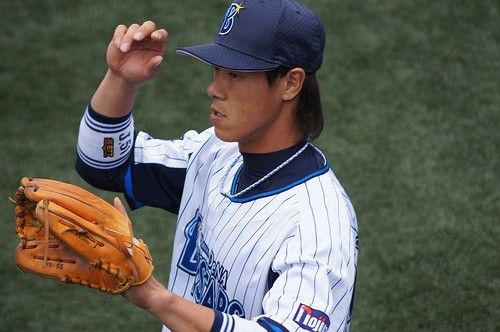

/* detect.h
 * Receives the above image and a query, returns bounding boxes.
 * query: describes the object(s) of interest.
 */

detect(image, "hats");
[176,0,325,73]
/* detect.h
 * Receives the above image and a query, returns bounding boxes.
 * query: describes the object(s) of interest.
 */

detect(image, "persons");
[75,0,360,332]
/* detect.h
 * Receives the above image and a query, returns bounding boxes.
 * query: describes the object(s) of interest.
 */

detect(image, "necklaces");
[219,141,310,198]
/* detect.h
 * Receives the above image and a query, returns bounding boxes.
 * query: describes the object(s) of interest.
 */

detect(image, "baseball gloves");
[8,177,154,295]
